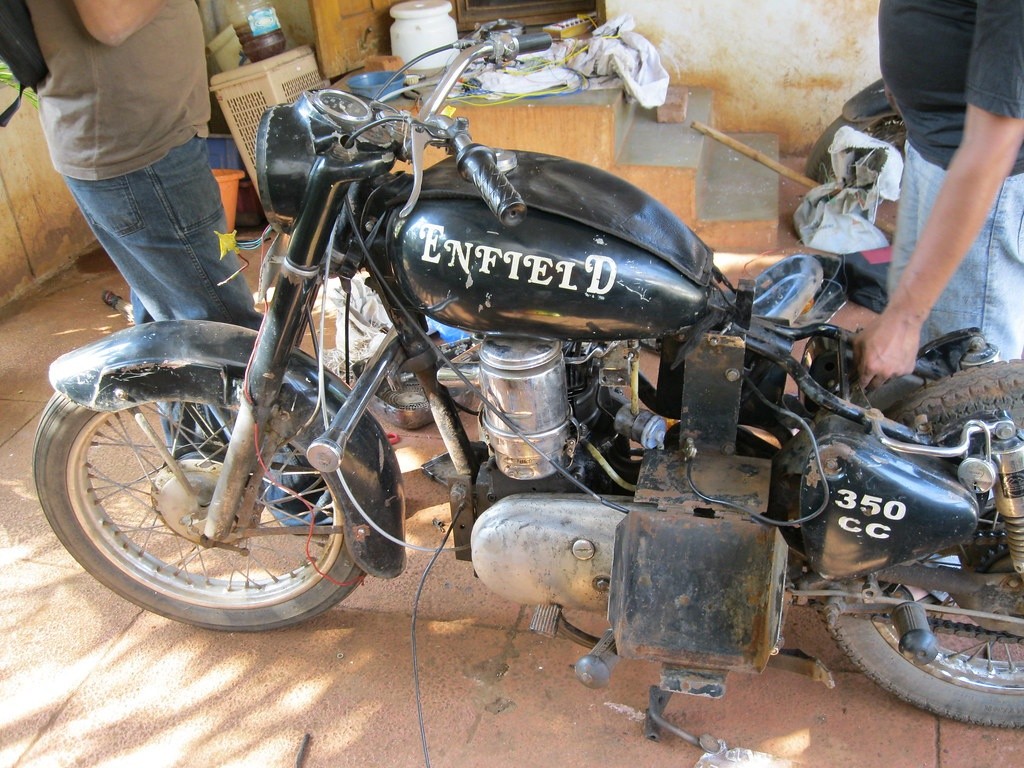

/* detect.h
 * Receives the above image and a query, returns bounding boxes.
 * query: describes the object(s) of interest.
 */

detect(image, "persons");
[26,0,335,526]
[854,0,1024,607]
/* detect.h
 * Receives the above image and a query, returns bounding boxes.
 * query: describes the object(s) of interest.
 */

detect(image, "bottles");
[223,0,286,62]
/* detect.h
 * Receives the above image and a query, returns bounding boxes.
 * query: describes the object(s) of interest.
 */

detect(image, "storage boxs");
[212,43,327,202]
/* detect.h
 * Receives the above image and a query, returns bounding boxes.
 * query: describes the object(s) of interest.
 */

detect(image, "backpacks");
[1,0,52,129]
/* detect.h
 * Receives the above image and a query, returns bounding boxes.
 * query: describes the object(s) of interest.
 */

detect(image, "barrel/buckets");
[209,168,245,234]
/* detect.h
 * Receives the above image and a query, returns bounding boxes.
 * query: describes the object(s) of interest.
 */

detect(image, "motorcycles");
[31,16,1024,731]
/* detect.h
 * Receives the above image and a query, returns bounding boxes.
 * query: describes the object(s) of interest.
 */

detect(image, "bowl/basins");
[345,71,406,102]
